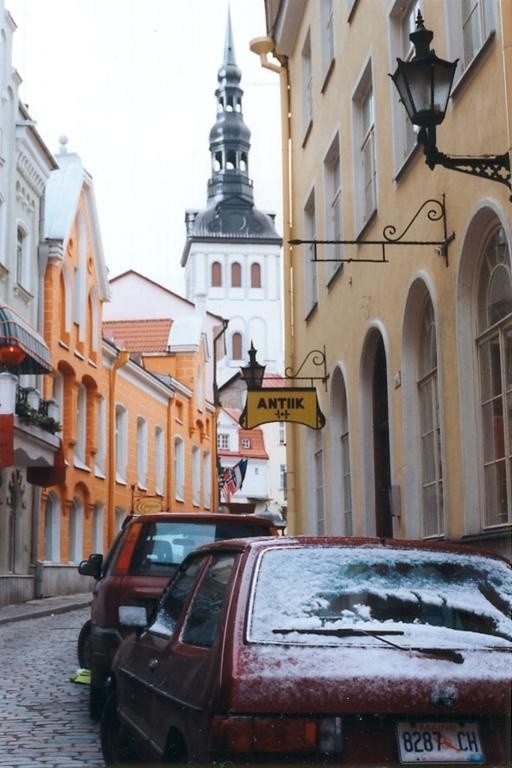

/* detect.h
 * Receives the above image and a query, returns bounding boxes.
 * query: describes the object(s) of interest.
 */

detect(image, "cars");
[103,535,511,761]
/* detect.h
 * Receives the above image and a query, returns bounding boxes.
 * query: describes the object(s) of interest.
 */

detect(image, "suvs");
[76,511,285,718]
